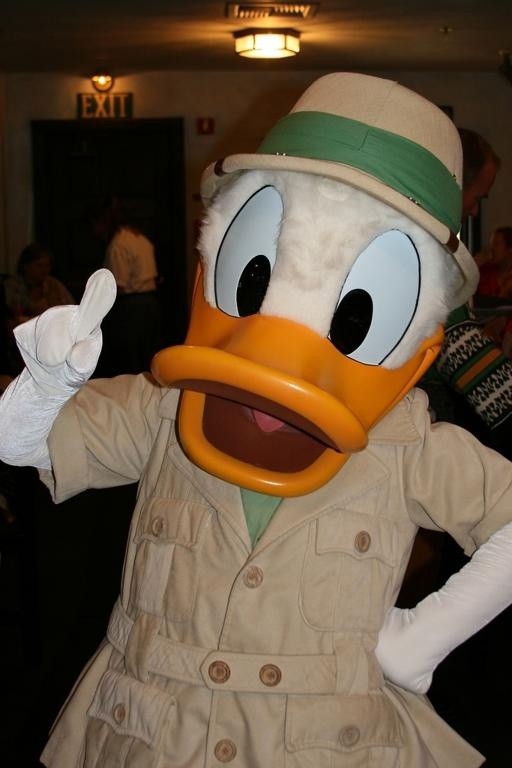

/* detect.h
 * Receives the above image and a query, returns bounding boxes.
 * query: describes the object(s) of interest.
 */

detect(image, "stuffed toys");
[1,70,511,766]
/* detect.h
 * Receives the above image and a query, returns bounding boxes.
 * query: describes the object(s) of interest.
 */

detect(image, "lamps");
[231,28,301,59]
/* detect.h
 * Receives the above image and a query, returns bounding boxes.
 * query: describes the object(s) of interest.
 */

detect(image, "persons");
[84,192,188,379]
[469,224,511,358]
[1,239,74,319]
[414,126,511,456]
